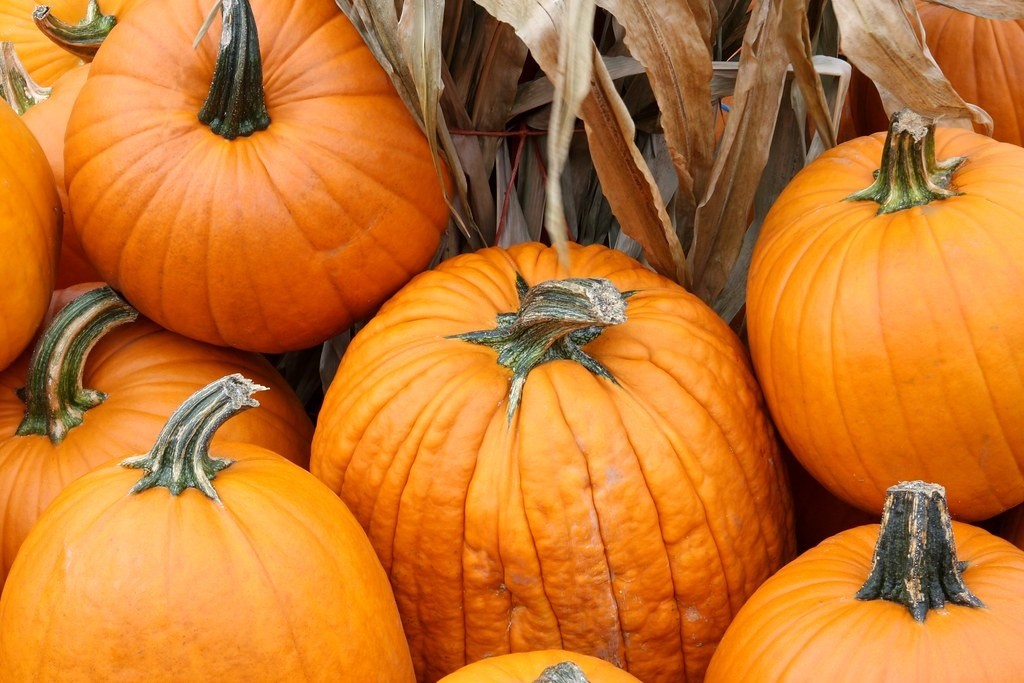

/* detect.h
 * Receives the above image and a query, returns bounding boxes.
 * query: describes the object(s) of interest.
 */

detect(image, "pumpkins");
[0,0,1024,683]
